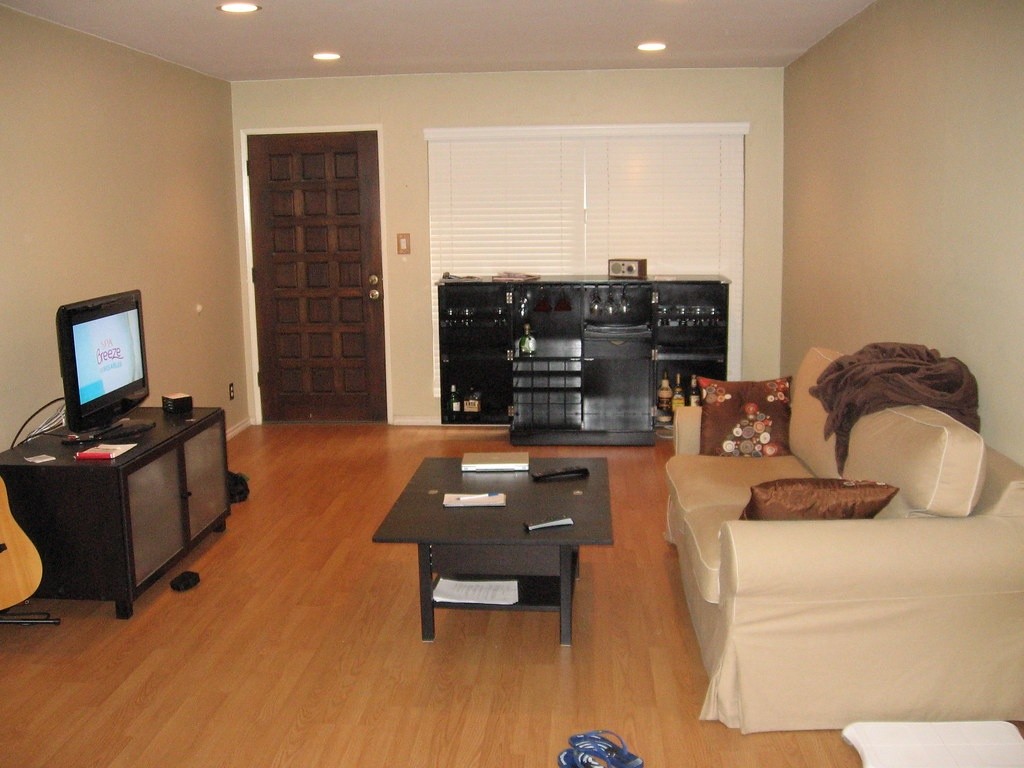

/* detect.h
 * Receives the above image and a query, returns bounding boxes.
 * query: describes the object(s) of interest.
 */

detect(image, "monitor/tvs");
[55,289,157,443]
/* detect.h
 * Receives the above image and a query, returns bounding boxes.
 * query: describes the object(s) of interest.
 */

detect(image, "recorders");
[607,258,647,278]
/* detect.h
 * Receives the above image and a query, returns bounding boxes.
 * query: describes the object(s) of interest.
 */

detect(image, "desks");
[0,407,230,620]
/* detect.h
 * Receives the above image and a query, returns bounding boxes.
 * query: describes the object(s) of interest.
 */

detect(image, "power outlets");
[229,382,235,400]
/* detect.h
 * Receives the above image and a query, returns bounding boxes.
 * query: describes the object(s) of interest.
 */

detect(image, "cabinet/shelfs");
[438,275,732,446]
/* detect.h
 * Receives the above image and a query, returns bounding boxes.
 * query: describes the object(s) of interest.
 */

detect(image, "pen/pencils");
[455,492,499,499]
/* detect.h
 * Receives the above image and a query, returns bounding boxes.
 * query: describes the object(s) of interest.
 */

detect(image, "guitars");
[0,476,43,612]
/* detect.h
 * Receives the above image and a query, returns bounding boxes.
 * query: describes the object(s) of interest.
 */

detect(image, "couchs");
[664,346,1024,733]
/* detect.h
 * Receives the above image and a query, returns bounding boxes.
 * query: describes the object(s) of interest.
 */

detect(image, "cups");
[446,309,456,325]
[461,309,473,324]
[495,308,505,324]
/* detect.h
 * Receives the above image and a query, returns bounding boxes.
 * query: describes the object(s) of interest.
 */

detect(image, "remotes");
[524,515,573,530]
[532,466,589,480]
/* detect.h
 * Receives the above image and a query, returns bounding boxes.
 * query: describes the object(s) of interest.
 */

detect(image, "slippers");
[558,748,605,768]
[568,730,644,768]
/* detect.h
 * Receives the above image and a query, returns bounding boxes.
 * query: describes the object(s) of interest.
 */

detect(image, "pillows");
[697,374,792,458]
[736,479,899,521]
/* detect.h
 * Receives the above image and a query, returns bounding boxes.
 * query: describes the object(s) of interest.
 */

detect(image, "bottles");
[463,387,482,423]
[658,371,672,422]
[659,308,670,326]
[708,308,717,325]
[692,307,702,326]
[520,324,537,355]
[448,385,461,422]
[688,375,700,406]
[672,373,687,423]
[678,308,687,326]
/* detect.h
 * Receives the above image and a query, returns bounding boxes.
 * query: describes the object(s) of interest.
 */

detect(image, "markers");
[75,451,115,460]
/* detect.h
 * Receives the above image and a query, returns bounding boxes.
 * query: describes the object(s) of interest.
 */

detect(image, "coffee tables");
[372,457,615,648]
